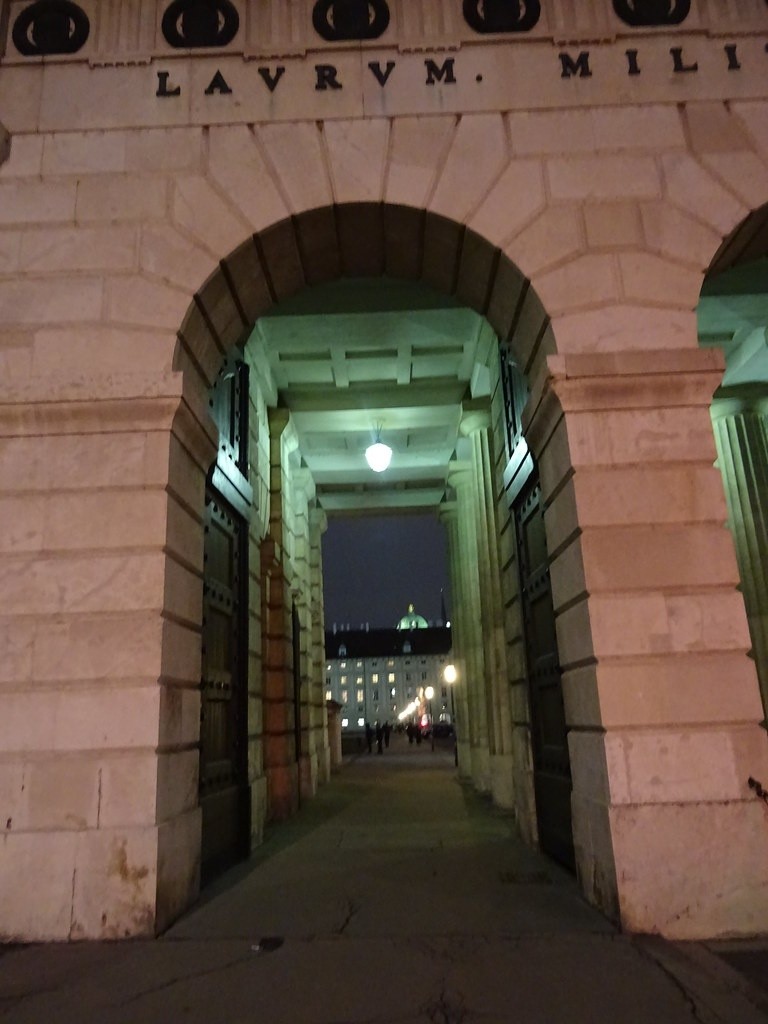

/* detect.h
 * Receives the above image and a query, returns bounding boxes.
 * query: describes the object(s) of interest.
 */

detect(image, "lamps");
[364,416,393,473]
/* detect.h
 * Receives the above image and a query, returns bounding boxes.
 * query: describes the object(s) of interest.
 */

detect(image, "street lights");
[424,686,437,751]
[443,665,459,768]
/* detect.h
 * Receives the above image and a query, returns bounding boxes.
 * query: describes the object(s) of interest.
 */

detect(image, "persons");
[365,722,376,754]
[382,720,391,748]
[407,722,422,745]
[376,724,384,755]
